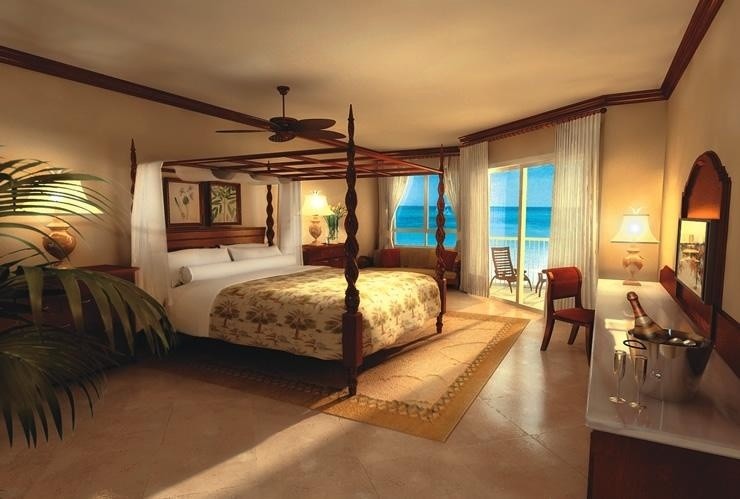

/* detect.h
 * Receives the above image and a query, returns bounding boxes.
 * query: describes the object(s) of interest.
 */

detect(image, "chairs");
[540,266,596,367]
[488,246,531,294]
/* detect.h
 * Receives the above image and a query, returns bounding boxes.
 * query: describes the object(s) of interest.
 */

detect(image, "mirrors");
[670,219,718,303]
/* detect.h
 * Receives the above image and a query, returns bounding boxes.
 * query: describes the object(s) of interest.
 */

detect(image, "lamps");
[17,168,106,273]
[297,191,336,243]
[608,206,661,287]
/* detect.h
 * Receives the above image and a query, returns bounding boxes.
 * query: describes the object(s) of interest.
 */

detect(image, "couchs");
[365,247,460,289]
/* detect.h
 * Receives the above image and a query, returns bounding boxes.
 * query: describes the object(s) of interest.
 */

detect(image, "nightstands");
[302,243,347,269]
[18,263,142,375]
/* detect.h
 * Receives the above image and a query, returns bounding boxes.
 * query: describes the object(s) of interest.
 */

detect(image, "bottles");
[627,292,665,341]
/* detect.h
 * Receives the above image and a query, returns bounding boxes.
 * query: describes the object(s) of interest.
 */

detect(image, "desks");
[584,276,740,499]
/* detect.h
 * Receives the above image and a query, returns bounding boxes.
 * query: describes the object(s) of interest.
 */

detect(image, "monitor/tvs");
[673,216,720,306]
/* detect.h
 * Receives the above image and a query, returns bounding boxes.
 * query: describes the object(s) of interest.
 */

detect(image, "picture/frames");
[163,177,207,228]
[207,181,243,226]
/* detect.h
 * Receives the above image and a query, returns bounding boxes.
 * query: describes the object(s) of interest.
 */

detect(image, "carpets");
[139,311,531,444]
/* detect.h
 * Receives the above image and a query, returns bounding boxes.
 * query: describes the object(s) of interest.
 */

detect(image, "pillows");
[160,248,233,287]
[380,248,400,268]
[444,251,458,272]
[227,245,283,263]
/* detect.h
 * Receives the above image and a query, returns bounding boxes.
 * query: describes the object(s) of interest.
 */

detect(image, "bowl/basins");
[623,328,714,402]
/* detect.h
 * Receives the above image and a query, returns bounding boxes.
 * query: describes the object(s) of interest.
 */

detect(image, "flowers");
[323,202,348,238]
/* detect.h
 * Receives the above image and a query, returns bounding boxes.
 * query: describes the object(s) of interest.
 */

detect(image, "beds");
[128,104,446,397]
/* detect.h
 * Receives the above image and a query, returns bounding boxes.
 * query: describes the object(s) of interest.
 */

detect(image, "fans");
[212,86,346,145]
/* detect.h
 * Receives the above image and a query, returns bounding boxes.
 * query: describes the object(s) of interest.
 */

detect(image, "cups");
[609,349,626,403]
[630,355,648,412]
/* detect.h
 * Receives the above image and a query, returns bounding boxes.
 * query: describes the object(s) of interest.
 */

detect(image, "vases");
[327,227,340,244]
